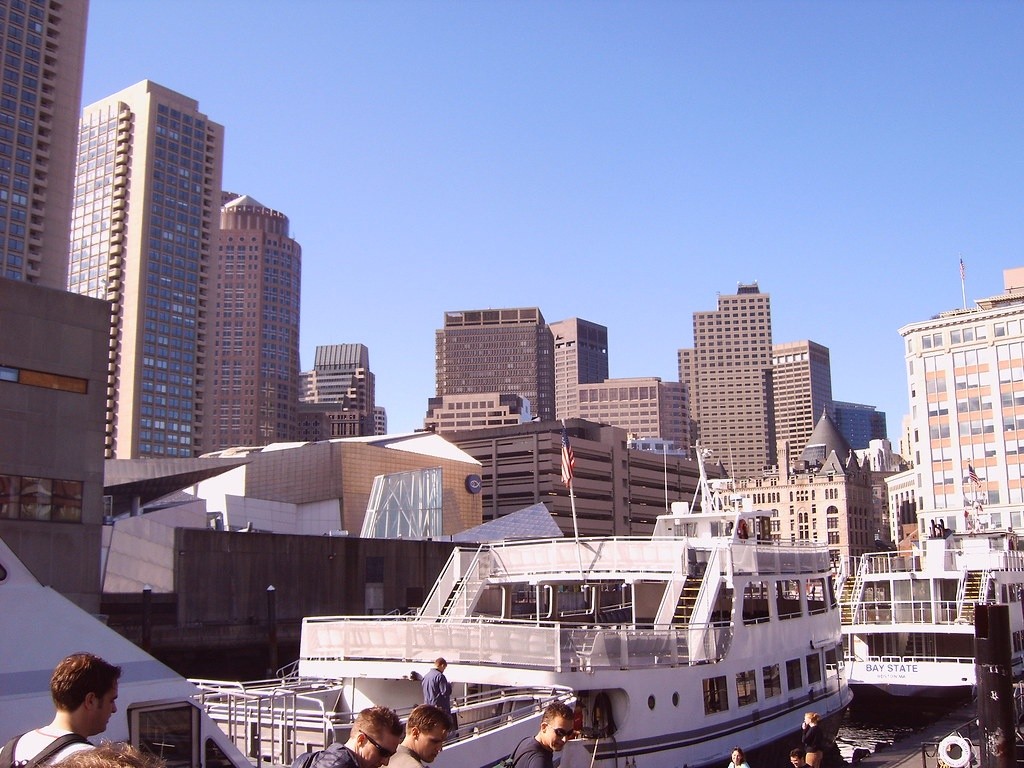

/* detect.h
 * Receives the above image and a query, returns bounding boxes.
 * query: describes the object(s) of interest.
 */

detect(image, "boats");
[1,418,1024,768]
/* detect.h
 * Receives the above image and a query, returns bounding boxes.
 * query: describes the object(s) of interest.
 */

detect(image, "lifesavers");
[937,734,972,768]
[739,519,749,539]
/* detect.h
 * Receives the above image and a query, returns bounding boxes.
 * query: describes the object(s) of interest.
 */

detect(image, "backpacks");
[492,735,552,768]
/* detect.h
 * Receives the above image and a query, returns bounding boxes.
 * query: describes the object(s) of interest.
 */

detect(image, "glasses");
[541,722,573,737]
[359,730,396,758]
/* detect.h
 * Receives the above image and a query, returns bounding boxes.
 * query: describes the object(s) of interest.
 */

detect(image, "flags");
[559,425,575,488]
[968,464,982,487]
[959,259,966,280]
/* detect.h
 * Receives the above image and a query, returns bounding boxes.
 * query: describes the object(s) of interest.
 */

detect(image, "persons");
[378,703,455,768]
[727,746,750,768]
[0,650,124,768]
[39,738,169,768]
[509,701,575,768]
[290,705,406,768]
[421,657,453,745]
[790,747,806,768]
[802,712,824,768]
